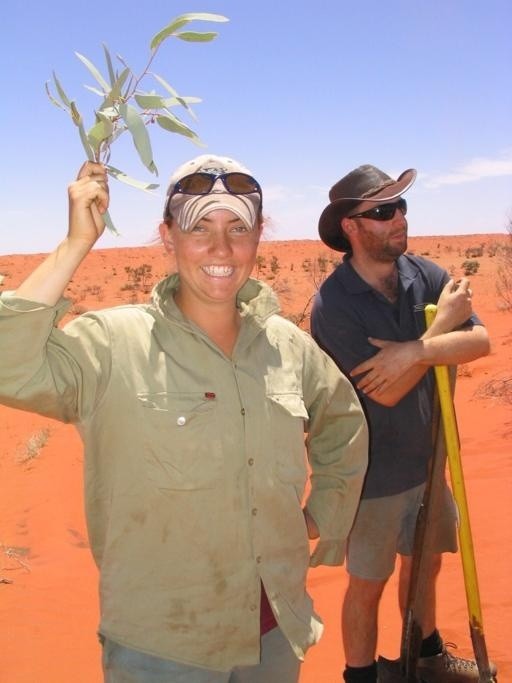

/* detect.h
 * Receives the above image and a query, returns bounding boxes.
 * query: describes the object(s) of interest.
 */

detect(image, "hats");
[165,155,261,234]
[318,166,415,251]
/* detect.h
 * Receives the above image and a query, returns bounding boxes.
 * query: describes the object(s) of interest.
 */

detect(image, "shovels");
[377,283,472,683]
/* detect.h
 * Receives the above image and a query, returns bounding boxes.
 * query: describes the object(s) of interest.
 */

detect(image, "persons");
[310,162,501,682]
[0,153,370,683]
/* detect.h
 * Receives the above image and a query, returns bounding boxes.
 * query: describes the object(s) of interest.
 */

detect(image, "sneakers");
[402,646,495,683]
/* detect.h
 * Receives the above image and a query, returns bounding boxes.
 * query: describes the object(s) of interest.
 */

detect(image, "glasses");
[346,199,408,223]
[166,173,262,221]
